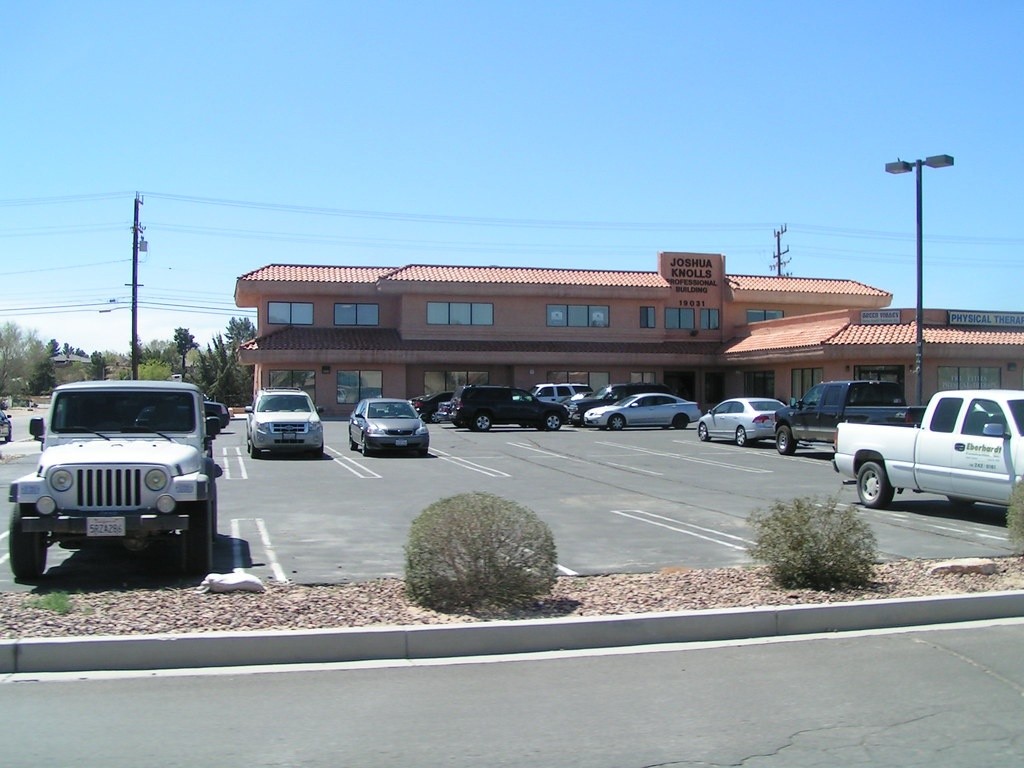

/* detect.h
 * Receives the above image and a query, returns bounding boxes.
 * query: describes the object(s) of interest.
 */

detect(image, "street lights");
[100,307,138,381]
[886,156,955,404]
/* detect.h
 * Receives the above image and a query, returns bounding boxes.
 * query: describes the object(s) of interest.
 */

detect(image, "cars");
[435,400,452,422]
[584,393,702,430]
[696,398,787,447]
[1,409,12,441]
[173,394,228,434]
[348,397,430,457]
[409,391,454,424]
[562,391,592,407]
[135,406,154,427]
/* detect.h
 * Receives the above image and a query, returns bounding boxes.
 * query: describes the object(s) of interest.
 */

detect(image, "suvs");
[4,379,224,578]
[453,384,570,431]
[522,383,593,408]
[244,386,324,458]
[565,382,677,425]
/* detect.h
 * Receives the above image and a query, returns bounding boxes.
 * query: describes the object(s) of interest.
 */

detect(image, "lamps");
[321,365,330,374]
[691,329,699,337]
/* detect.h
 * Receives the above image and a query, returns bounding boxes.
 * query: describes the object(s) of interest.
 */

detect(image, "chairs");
[968,410,990,434]
[369,407,377,418]
[150,401,182,431]
[376,409,385,417]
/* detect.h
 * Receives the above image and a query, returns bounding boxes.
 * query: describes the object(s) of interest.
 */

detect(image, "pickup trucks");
[832,389,1024,510]
[773,380,927,457]
